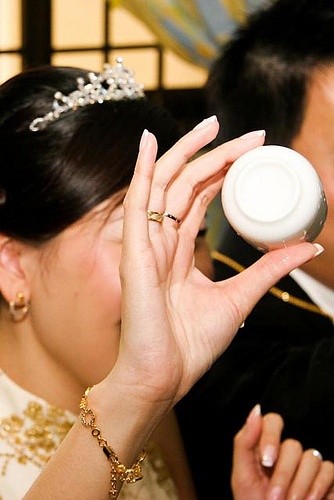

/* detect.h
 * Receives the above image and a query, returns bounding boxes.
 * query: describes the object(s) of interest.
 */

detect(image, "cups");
[222,144,327,253]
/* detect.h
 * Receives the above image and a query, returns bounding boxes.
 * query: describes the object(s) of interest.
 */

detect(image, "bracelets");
[78,385,147,500]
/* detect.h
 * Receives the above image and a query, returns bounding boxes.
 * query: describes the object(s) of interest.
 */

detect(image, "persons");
[170,0,334,500]
[0,64,334,495]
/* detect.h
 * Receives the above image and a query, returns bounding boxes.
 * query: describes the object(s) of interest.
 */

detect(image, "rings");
[147,210,165,223]
[164,211,182,225]
